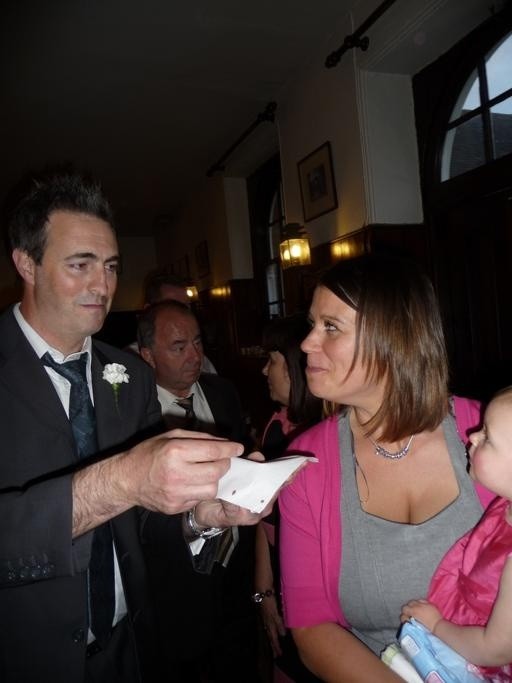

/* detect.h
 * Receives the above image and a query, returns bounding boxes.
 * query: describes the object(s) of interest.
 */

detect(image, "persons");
[127,306,256,681]
[125,266,218,374]
[396,385,512,682]
[0,178,273,681]
[255,333,329,681]
[276,256,499,683]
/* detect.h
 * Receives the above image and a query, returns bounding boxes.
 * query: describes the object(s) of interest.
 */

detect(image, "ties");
[42,352,117,642]
[174,394,199,421]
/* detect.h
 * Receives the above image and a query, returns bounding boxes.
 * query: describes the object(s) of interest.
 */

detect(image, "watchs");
[187,510,228,541]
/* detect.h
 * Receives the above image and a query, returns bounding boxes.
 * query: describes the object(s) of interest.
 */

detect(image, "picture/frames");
[294,141,339,222]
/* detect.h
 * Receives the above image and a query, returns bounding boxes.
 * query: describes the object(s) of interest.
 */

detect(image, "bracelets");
[251,587,273,605]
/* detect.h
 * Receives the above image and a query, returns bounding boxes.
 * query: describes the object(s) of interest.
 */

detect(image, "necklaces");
[358,425,414,459]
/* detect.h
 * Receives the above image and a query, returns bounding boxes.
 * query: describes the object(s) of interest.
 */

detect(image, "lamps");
[278,223,313,313]
